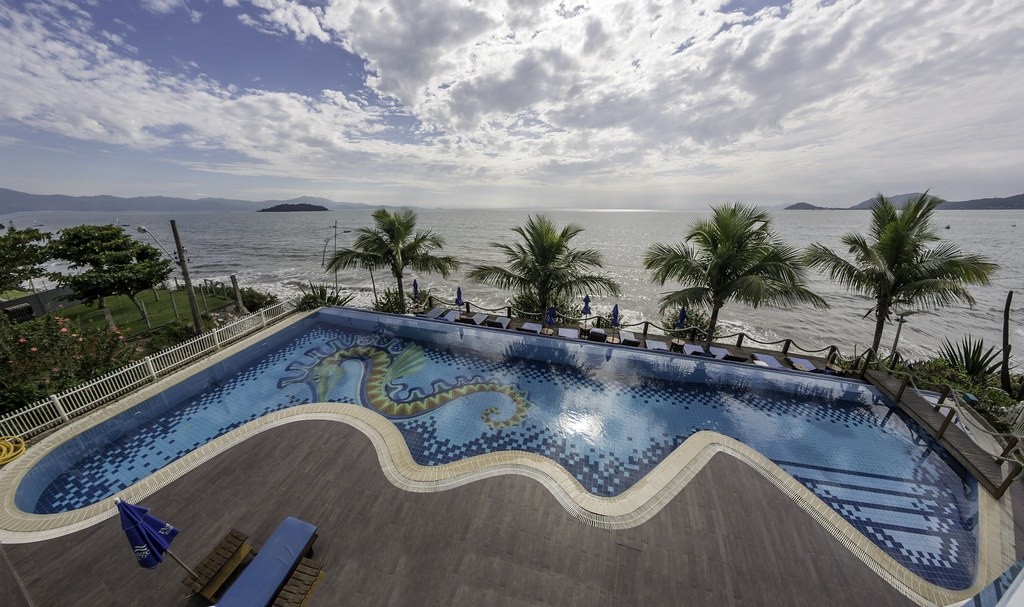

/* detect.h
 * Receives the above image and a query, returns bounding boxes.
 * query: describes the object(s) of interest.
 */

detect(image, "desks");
[541,327,554,335]
[213,516,318,607]
[670,339,685,353]
[606,336,620,344]
[827,363,841,372]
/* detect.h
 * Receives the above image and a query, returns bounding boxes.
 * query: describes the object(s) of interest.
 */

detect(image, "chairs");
[645,340,668,350]
[590,327,607,343]
[710,346,748,362]
[683,343,710,358]
[437,310,462,322]
[460,313,489,326]
[751,352,783,368]
[416,306,447,320]
[517,322,542,334]
[786,356,825,373]
[619,330,641,347]
[557,327,579,340]
[487,316,511,330]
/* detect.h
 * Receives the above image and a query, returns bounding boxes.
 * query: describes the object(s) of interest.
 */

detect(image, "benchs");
[271,558,324,607]
[180,529,257,603]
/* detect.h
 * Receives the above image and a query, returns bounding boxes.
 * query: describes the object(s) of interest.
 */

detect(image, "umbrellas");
[675,306,687,343]
[543,307,555,333]
[413,279,419,311]
[611,304,619,341]
[115,498,198,579]
[455,286,464,319]
[581,295,591,329]
